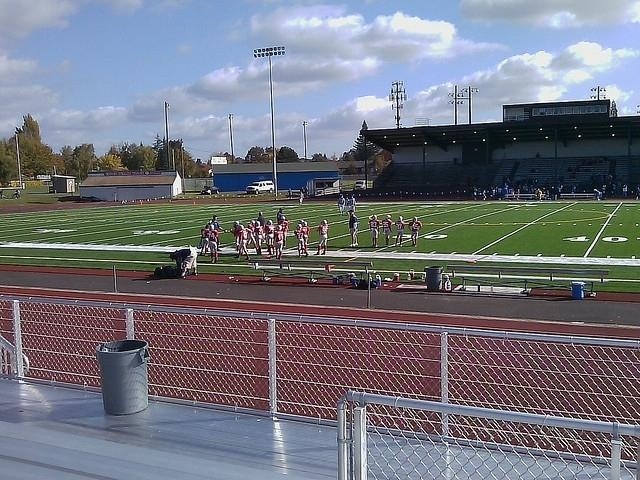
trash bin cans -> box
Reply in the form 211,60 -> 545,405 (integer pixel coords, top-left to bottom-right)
96,339 -> 152,415
424,265 -> 443,291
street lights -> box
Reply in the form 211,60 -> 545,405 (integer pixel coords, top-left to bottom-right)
228,113 -> 234,163
389,80 -> 407,128
253,45 -> 286,197
301,120 -> 308,159
165,101 -> 170,170
447,85 -> 480,125
590,85 -> 607,100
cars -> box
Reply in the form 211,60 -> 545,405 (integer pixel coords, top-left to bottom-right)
246,180 -> 274,195
201,186 -> 220,195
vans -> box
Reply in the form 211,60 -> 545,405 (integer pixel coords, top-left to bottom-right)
355,180 -> 367,190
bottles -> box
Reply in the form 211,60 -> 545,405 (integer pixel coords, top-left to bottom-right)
394,273 -> 399,281
409,269 -> 414,278
376,275 -> 382,287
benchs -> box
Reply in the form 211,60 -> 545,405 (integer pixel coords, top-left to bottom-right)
249,258 -> 373,284
444,264 -> 612,295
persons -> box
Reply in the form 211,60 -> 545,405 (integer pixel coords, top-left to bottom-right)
348,210 -> 422,247
338,194 -> 356,216
536,152 -> 540,158
582,156 -> 609,166
200,208 -> 328,264
0,189 -> 20,200
169,248 -> 198,279
298,191 -> 304,206
473,166 -> 640,202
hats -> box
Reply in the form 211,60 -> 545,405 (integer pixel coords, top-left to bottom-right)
170,250 -> 177,262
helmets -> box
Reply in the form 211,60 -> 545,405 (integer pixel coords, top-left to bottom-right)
232,219 -> 327,230
371,214 -> 418,221
